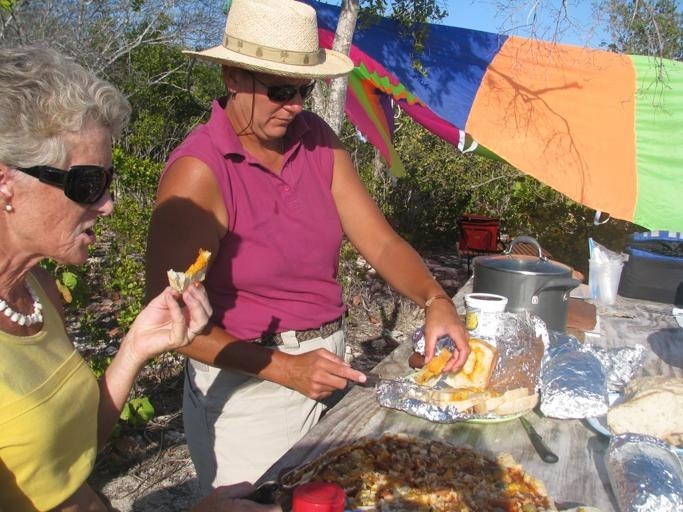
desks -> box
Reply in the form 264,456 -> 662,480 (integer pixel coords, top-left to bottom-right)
254,275 -> 683,512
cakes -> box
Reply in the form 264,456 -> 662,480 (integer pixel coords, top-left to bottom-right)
284,433 -> 559,511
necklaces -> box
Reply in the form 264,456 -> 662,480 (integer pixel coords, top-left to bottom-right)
1,278 -> 43,327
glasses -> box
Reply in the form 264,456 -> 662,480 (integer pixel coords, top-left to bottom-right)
245,69 -> 316,103
2,158 -> 114,205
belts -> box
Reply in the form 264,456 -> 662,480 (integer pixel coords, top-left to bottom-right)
240,310 -> 349,346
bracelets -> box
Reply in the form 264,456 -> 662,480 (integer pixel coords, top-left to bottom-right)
423,294 -> 455,315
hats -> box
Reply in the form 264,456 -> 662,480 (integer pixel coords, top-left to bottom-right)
181,0 -> 355,81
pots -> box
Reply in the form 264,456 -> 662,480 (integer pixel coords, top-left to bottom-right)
472,234 -> 581,333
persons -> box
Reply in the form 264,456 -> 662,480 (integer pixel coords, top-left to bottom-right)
143,0 -> 472,495
1,37 -> 286,512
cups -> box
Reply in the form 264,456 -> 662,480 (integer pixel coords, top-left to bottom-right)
463,293 -> 508,336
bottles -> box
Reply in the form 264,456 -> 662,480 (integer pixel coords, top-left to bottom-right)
291,486 -> 346,512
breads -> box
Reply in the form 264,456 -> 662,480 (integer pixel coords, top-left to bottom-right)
605,373 -> 683,443
164,248 -> 211,293
409,336 -> 538,419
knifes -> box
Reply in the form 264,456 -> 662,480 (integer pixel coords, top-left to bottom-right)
520,415 -> 559,463
